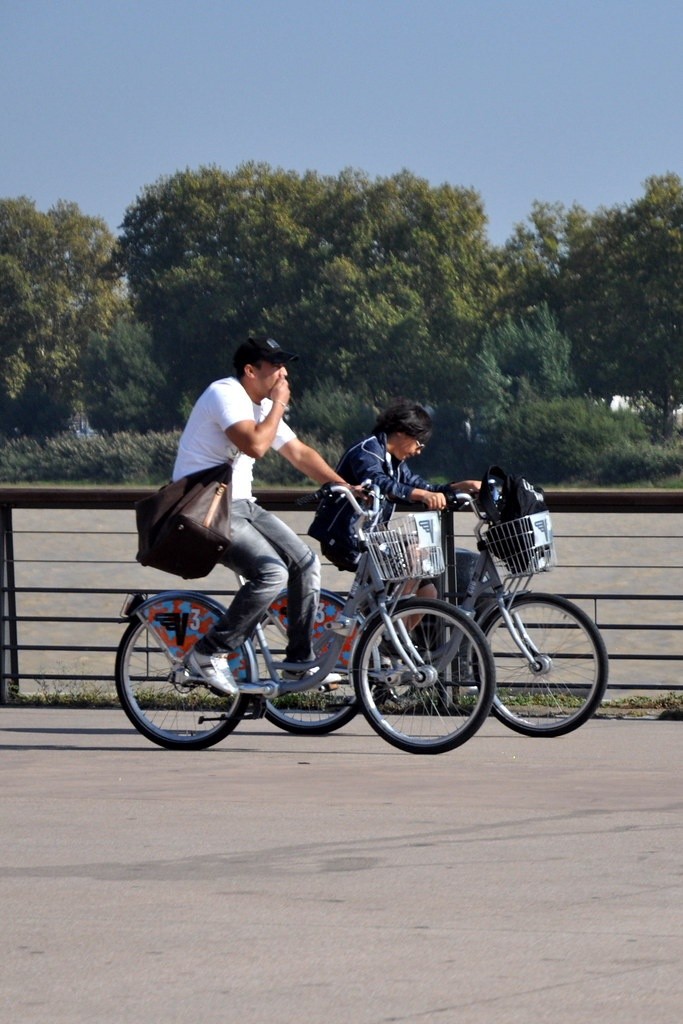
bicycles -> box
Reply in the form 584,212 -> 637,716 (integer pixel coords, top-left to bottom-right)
251,479 -> 611,736
112,479 -> 496,754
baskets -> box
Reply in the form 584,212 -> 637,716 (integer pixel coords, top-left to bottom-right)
360,512 -> 445,581
482,510 -> 557,576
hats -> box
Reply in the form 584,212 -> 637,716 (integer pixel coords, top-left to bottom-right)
234,339 -> 300,364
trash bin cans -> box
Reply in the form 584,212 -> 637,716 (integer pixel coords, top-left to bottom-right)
379,547 -> 479,686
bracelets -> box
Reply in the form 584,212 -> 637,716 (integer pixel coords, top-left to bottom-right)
274,400 -> 286,408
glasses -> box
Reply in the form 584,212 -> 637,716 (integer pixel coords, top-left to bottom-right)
412,438 -> 426,452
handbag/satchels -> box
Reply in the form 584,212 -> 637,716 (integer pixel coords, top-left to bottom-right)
135,462 -> 232,578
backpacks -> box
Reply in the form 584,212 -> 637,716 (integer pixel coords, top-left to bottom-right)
482,467 -> 544,571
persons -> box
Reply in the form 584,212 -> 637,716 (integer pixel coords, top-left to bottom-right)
307,404 -> 485,682
172,333 -> 369,696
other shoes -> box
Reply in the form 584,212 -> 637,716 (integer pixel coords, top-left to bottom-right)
379,635 -> 428,661
373,685 -> 405,705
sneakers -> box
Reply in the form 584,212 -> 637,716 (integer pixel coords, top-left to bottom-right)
280,662 -> 341,687
185,647 -> 238,697
